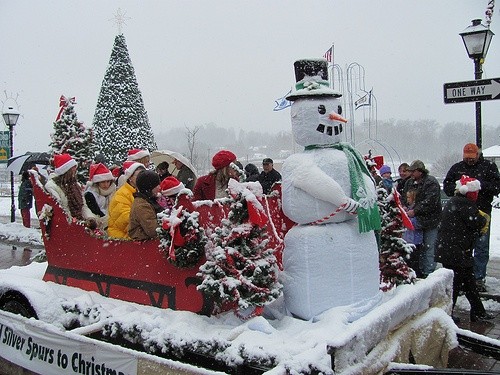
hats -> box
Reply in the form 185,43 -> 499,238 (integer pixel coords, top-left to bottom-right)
462,143 -> 477,159
380,165 -> 391,176
112,168 -> 120,181
284,58 -> 343,101
406,159 -> 425,171
89,163 -> 114,183
157,161 -> 169,170
161,176 -> 185,197
212,150 -> 236,170
123,161 -> 146,179
263,158 -> 273,166
455,175 -> 481,195
128,149 -> 150,160
136,170 -> 163,194
54,154 -> 78,176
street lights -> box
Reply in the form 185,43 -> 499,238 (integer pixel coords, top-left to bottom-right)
1,106 -> 21,223
458,19 -> 495,154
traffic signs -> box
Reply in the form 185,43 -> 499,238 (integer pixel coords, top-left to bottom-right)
443,77 -> 500,104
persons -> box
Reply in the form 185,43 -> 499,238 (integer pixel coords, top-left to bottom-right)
281,59 -> 379,322
366,144 -> 500,322
19,149 -> 282,242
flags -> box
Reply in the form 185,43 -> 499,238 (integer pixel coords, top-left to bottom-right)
273,91 -> 291,111
322,47 -> 333,62
354,91 -> 372,110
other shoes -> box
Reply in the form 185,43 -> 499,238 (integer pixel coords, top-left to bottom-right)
475,280 -> 487,292
416,272 -> 428,279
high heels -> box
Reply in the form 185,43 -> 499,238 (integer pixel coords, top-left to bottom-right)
451,316 -> 460,323
470,308 -> 495,322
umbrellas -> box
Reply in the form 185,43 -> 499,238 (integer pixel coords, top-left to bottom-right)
149,150 -> 198,178
6,153 -> 53,174
